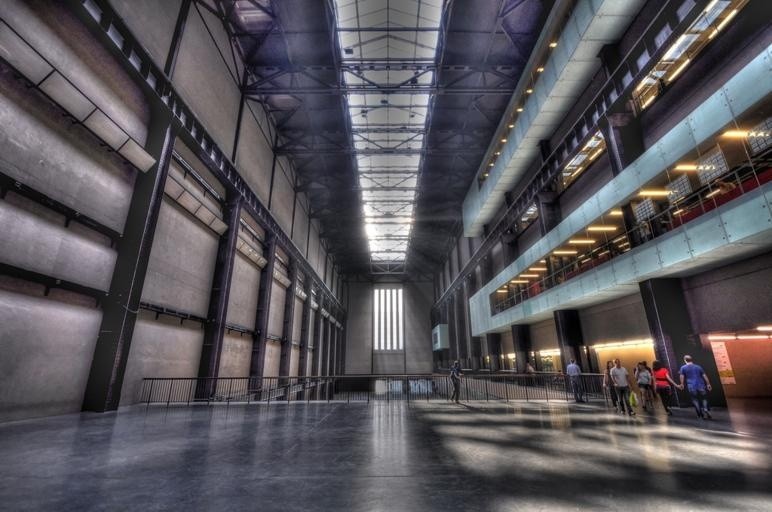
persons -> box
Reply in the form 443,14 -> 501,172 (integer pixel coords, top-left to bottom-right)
657,77 -> 670,95
679,355 -> 712,420
633,220 -> 651,243
652,360 -> 682,416
567,359 -> 586,403
449,361 -> 464,404
715,178 -> 736,195
525,363 -> 534,374
602,358 -> 656,416
530,280 -> 540,296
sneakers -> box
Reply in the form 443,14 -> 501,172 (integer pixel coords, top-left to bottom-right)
576,398 -> 584,403
697,408 -> 712,420
613,404 -> 673,416
